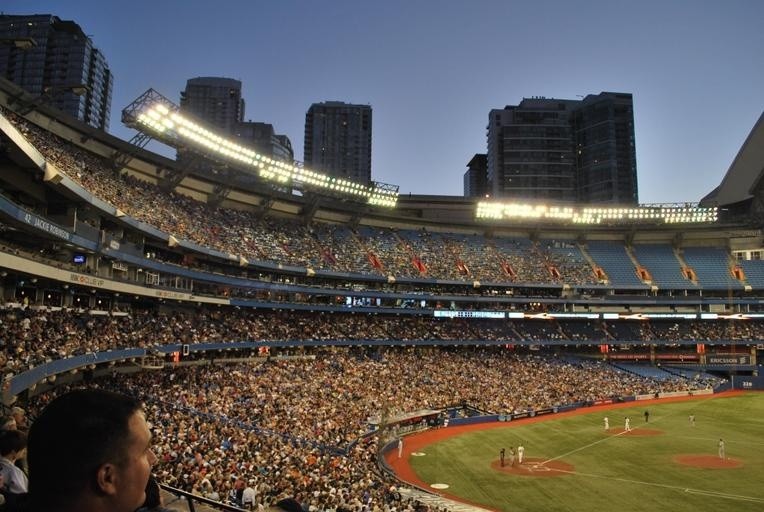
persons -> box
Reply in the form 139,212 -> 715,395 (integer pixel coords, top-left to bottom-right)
2,102 -> 764,511
